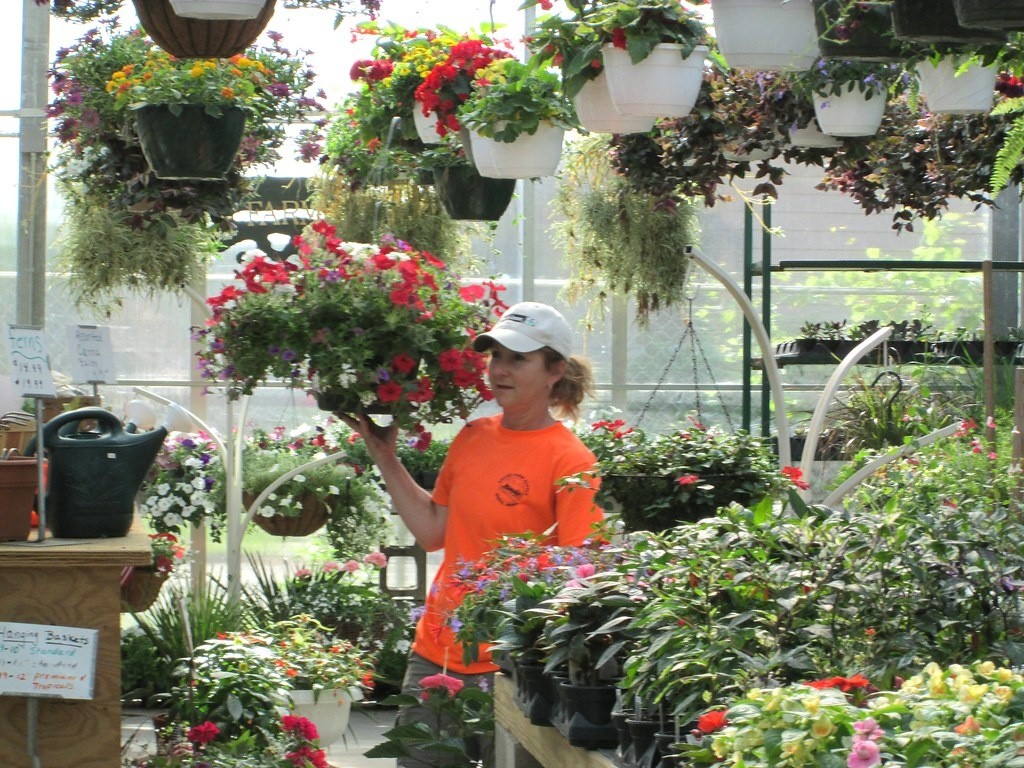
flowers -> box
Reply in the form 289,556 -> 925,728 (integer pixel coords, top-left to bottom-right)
348,18 -> 506,140
573,69 -> 657,134
362,673 -> 497,768
561,0 -> 731,76
786,56 -> 912,110
753,70 -> 822,134
146,531 -> 200,581
414,39 -> 521,138
189,219 -> 510,452
285,552 -> 387,638
105,49 -> 275,119
415,132 -> 468,172
209,446 -> 400,561
41,21 -> 331,175
554,405 -> 811,519
223,414 -> 454,475
389,520 -> 659,684
455,57 -> 589,143
519,14 -> 603,101
137,632 -> 336,768
660,417 -> 1024,768
136,433 -> 215,536
214,613 -> 387,707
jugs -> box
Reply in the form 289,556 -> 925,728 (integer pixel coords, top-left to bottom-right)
22,406 -> 167,539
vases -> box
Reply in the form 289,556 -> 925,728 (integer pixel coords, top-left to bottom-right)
509,647 -> 709,768
309,332 -> 422,414
126,175 -> 176,212
411,99 -> 441,143
464,119 -> 566,178
788,110 -> 844,148
609,473 -> 753,530
712,0 -> 819,72
917,52 -> 997,114
600,42 -> 709,119
953,0 -> 1024,30
288,688 -> 351,748
0,395 -> 102,543
721,136 -> 774,161
813,0 -> 916,63
131,0 -> 277,58
169,0 -> 266,20
811,79 -> 888,137
136,103 -> 246,180
431,164 -> 515,221
891,0 -> 1008,45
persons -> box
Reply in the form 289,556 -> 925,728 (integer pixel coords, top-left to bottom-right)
331,301 -> 612,768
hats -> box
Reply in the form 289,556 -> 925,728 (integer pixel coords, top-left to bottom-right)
475,302 -> 573,362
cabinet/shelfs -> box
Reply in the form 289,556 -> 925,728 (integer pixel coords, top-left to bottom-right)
741,195 -> 1024,463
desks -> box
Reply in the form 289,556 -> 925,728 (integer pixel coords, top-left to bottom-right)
0,500 -> 153,768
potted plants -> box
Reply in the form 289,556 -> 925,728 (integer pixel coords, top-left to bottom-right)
794,321 -> 821,352
909,320 -> 933,355
819,318 -> 847,355
933,324 -> 1024,366
881,320 -> 914,356
846,320 -> 881,358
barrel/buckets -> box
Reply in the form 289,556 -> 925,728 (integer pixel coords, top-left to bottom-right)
0,456 -> 42,543
